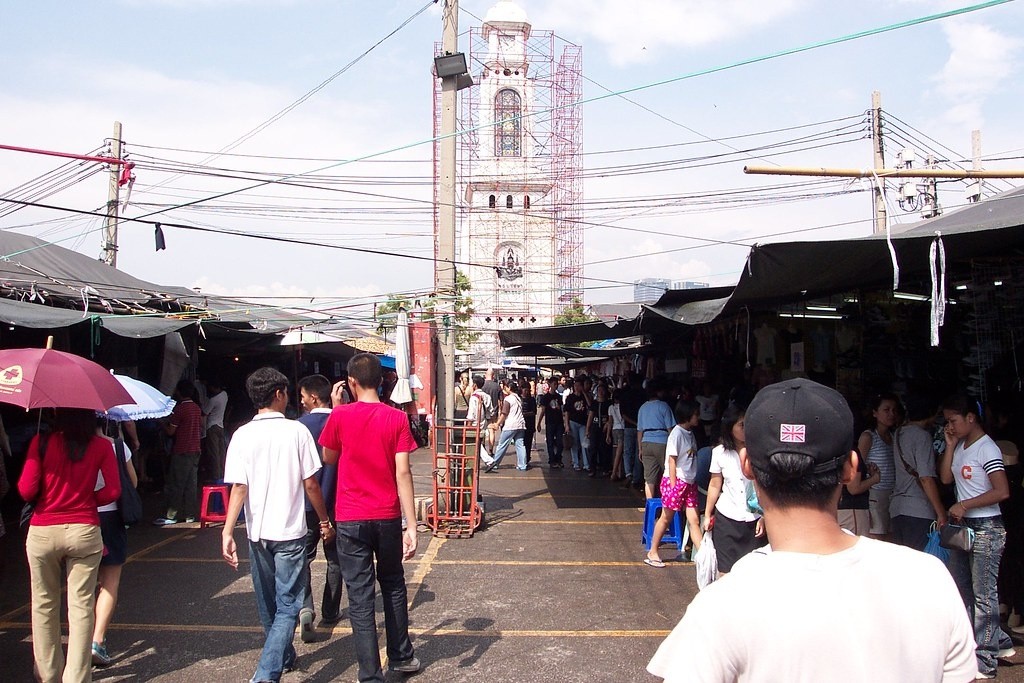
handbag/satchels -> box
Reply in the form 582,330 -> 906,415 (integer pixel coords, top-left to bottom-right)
938,516 -> 976,552
117,437 -> 144,524
924,520 -> 969,584
563,431 -> 573,449
694,531 -> 719,592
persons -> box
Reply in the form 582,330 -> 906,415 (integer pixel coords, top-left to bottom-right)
91,409 -> 142,666
151,379 -> 203,527
297,374 -> 342,643
318,351 -> 427,683
754,322 -> 858,371
201,377 -> 229,485
16,405 -> 122,683
646,376 -> 981,683
836,392 -> 1018,682
221,367 -> 337,683
448,373 -> 769,579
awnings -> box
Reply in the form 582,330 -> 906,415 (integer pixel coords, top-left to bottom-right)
491,186 -> 1024,417
0,226 -> 366,364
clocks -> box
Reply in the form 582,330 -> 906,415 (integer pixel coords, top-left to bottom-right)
500,37 -> 515,51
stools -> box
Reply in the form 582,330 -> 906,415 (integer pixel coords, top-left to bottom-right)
200,486 -> 230,529
641,499 -> 682,553
214,479 -> 246,524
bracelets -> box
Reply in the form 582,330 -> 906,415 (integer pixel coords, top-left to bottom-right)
318,517 -> 331,528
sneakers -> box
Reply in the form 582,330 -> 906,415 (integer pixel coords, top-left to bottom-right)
92,636 -> 110,664
389,658 -> 420,670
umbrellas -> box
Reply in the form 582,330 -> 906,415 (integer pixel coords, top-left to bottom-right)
390,302 -> 415,413
0,336 -> 180,437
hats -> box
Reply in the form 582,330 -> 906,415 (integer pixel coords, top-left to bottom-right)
743,378 -> 853,475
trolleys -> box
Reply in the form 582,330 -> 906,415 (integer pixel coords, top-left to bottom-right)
426,395 -> 482,536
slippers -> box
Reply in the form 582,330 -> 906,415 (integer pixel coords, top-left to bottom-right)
644,559 -> 666,568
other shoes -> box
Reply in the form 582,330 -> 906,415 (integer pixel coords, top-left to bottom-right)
998,647 -> 1016,658
323,611 -> 344,624
299,612 -> 316,642
975,672 -> 995,679
552,462 -> 633,489
484,462 -> 496,473
153,518 -> 176,525
186,517 -> 194,523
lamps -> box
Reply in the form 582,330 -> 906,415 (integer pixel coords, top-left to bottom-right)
954,278 -> 1003,290
806,302 -> 839,312
843,295 -> 924,306
893,292 -> 957,305
776,309 -> 842,320
